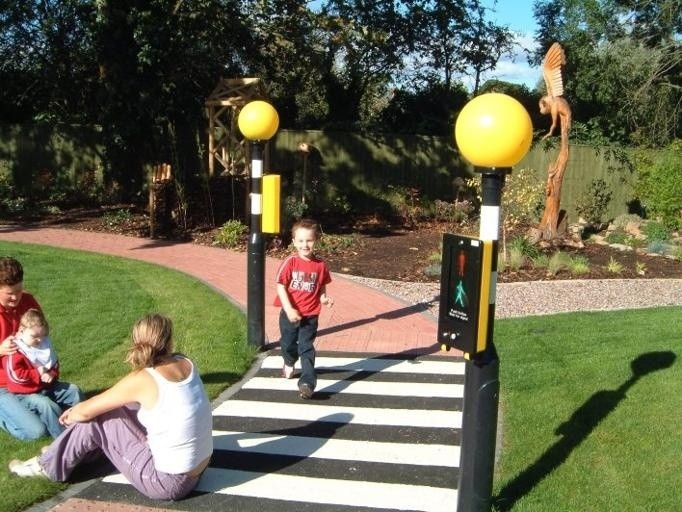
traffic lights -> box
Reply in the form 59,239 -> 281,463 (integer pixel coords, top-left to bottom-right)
436,232 -> 487,359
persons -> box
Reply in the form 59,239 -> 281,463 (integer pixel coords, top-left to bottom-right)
272,218 -> 334,400
0,256 -> 82,443
9,313 -> 214,502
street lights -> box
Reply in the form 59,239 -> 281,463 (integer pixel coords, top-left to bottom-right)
234,98 -> 280,350
451,88 -> 538,504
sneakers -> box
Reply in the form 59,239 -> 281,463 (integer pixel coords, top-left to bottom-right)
283,364 -> 295,379
297,377 -> 315,400
8,444 -> 52,479
